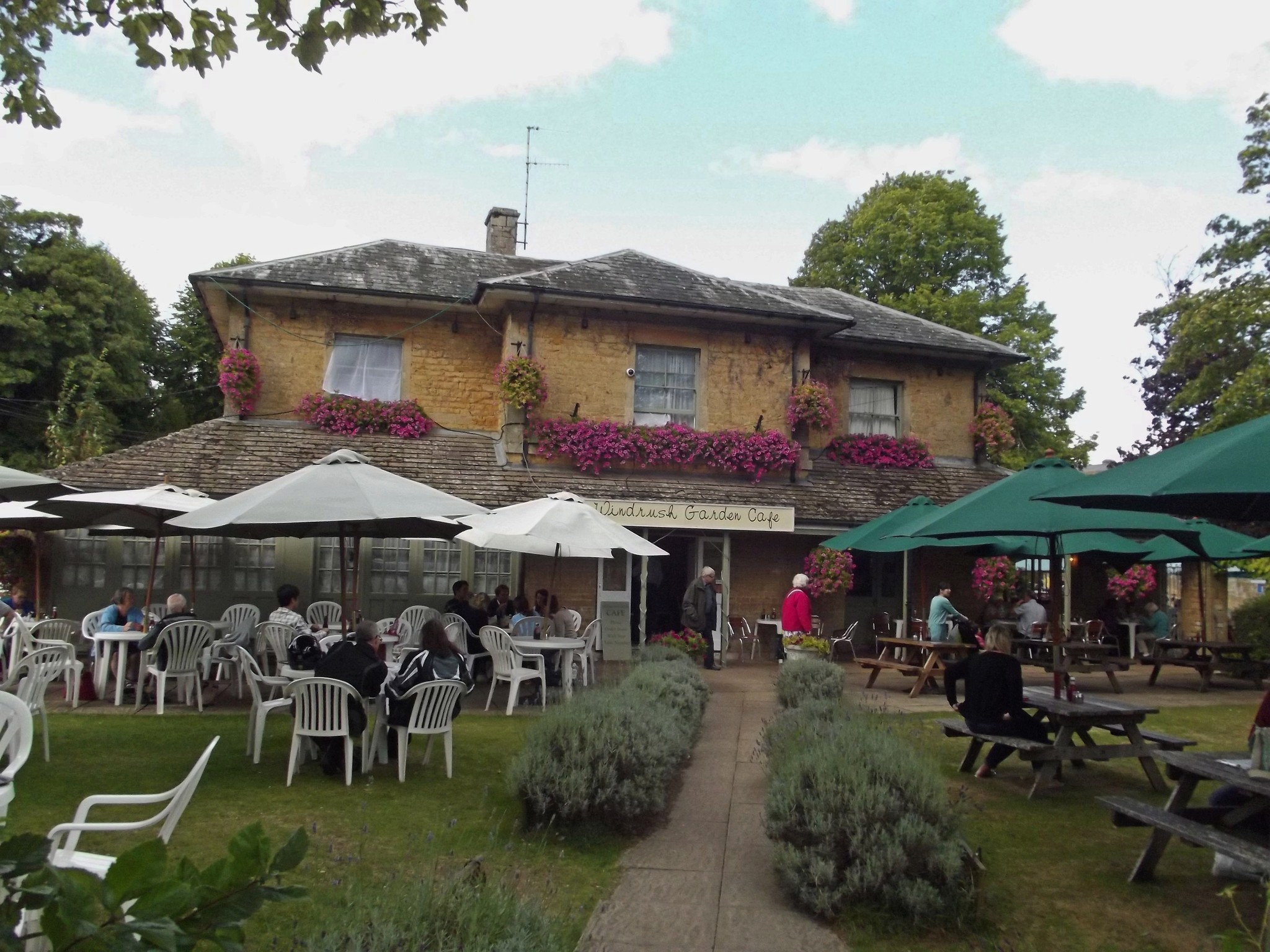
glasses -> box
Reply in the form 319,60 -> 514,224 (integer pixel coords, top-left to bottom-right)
376,635 -> 383,641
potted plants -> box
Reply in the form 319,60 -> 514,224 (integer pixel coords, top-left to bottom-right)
782,634 -> 829,662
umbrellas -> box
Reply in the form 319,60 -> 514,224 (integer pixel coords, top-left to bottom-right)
269,519 -> 472,609
1229,535 -> 1270,554
455,491 -> 670,641
818,496 -> 1029,660
162,449 -> 491,642
0,466 -> 219,633
878,410 -> 1270,772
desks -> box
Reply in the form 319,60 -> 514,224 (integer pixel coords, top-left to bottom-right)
0,619 -> 81,677
861,638 -> 976,698
893,619 -> 955,659
1129,748 -> 1270,883
1119,621 -> 1137,658
511,636 -> 585,701
93,631 -> 146,705
279,658 -> 401,765
749,619 -> 782,661
991,619 -> 1021,656
1150,638 -> 1264,693
204,619 -> 232,680
1009,639 -> 1123,695
811,615 -> 1121,663
379,635 -> 399,661
960,686 -> 1167,797
307,621 -> 348,630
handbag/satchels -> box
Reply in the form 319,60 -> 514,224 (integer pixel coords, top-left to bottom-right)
64,671 -> 96,700
287,633 -> 321,669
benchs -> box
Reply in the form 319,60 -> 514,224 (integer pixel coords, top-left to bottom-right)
859,637 -> 1270,883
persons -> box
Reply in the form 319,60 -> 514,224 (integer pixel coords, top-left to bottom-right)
681,566 -> 722,670
1135,600 -> 1170,657
929,582 -> 980,670
0,584 -> 36,676
943,624 -> 1040,777
781,574 -> 812,653
1208,689 -> 1270,796
1004,576 -> 1047,657
100,588 -> 209,703
445,581 -> 578,685
269,585 -> 329,641
1098,599 -> 1129,656
290,619 -> 474,772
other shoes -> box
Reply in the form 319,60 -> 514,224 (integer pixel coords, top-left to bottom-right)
704,663 -> 721,670
317,751 -> 337,773
125,682 -> 134,690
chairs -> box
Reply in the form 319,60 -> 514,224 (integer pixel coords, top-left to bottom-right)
0,690 -> 219,952
725,615 -> 761,662
1,601 -> 601,787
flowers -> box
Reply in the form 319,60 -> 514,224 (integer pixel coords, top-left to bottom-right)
652,629 -> 706,661
218,346 -> 1016,482
803,547 -> 1157,595
